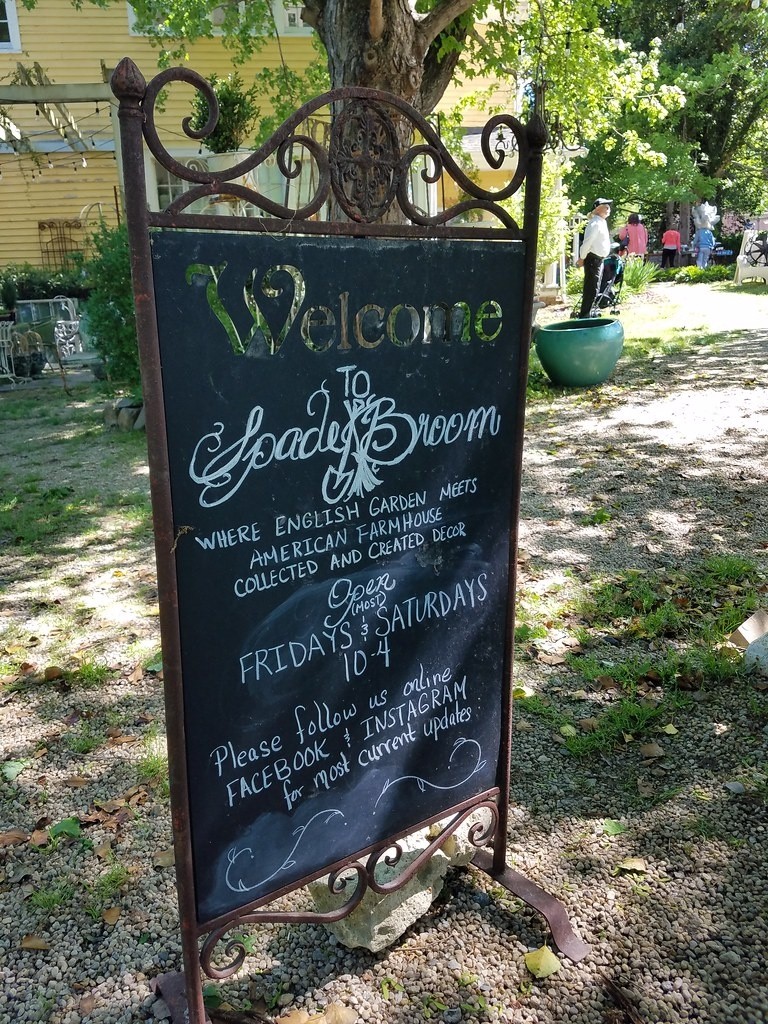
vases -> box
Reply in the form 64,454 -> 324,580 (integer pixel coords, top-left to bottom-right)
534,318 -> 624,388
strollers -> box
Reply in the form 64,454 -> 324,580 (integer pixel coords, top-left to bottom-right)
570,232 -> 629,318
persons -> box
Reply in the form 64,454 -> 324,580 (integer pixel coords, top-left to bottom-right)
693,228 -> 720,271
619,214 -> 648,261
660,224 -> 680,268
577,198 -> 613,319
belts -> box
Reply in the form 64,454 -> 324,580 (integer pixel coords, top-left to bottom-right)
589,253 -> 602,260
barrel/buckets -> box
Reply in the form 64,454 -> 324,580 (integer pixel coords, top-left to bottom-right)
534,316 -> 625,386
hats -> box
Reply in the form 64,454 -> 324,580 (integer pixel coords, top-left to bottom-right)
593,198 -> 613,209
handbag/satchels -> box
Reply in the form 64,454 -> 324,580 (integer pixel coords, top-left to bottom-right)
620,237 -> 629,250
694,247 -> 699,254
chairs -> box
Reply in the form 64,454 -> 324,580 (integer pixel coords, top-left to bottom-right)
734,229 -> 768,286
